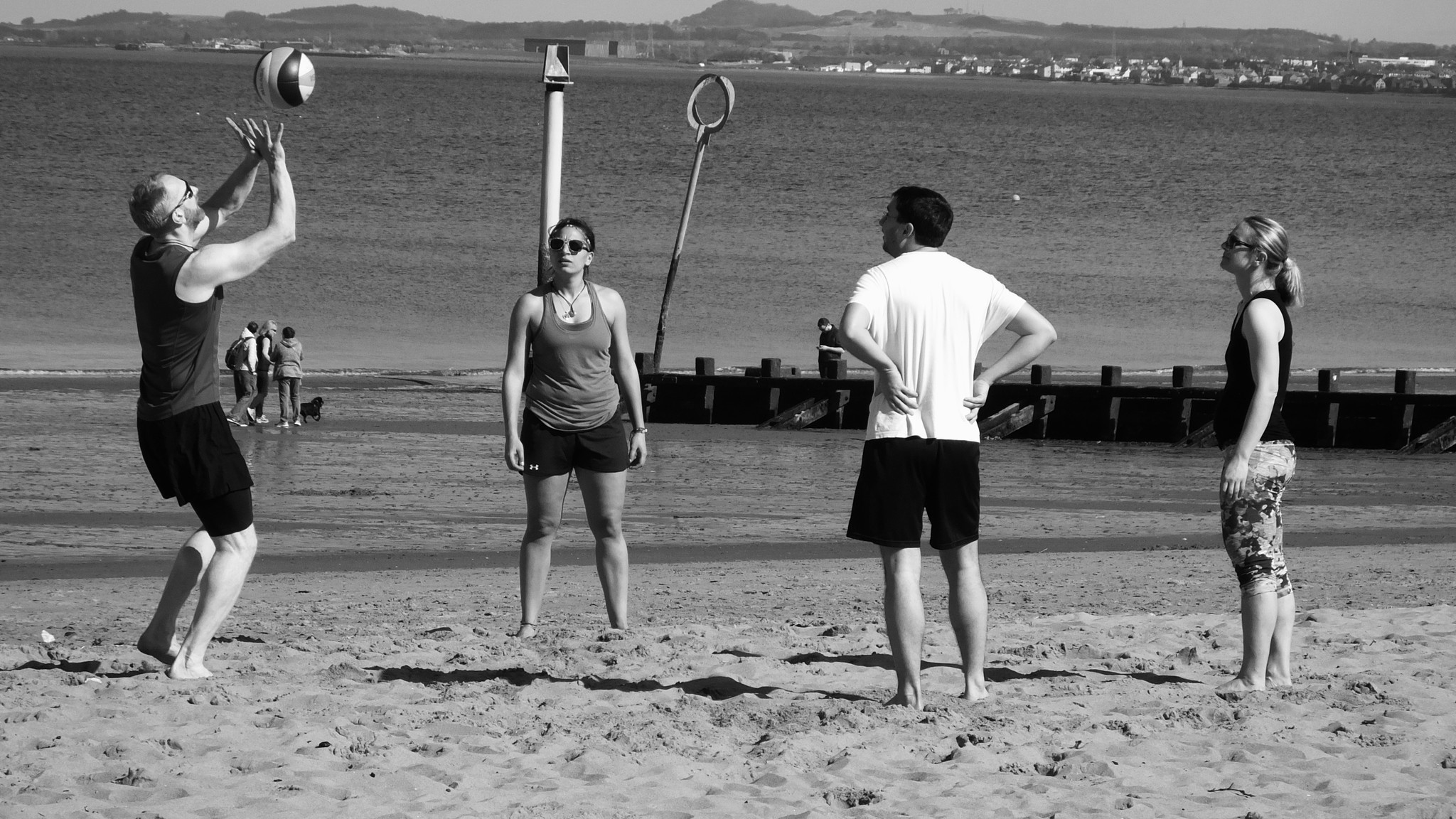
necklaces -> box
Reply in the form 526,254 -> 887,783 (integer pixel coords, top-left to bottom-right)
550,284 -> 586,319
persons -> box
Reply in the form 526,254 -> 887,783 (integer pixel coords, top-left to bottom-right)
815,319 -> 846,379
226,320 -> 304,428
502,218 -> 648,638
836,184 -> 1060,710
129,116 -> 297,683
1219,214 -> 1306,694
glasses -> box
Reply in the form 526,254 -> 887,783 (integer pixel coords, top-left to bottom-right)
273,329 -> 276,332
162,178 -> 193,222
548,237 -> 591,252
819,323 -> 828,331
1226,233 -> 1262,254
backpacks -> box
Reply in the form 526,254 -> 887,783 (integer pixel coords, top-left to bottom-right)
225,337 -> 256,371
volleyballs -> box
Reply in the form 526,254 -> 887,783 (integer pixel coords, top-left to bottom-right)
252,45 -> 316,111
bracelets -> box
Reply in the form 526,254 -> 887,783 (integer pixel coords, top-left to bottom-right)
629,427 -> 648,441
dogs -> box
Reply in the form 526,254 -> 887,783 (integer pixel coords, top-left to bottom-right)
300,396 -> 324,423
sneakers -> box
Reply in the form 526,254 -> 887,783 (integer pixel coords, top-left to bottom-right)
247,407 -> 255,421
255,414 -> 269,423
226,415 -> 249,426
274,422 -> 289,428
294,421 -> 301,426
